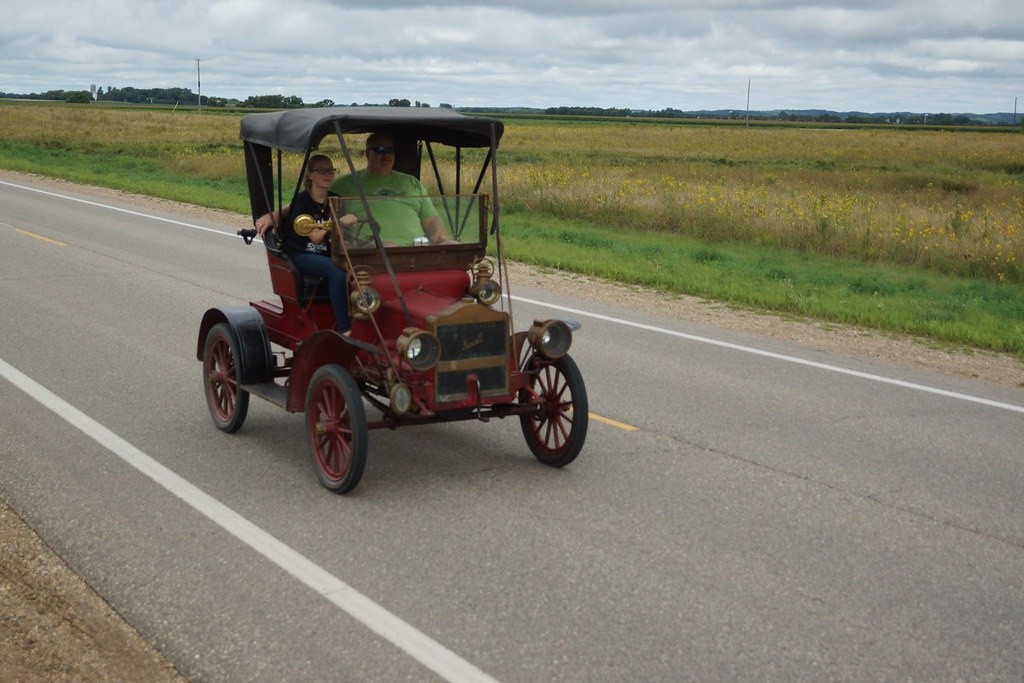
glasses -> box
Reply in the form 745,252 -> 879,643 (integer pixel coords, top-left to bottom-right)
369,145 -> 396,154
312,167 -> 336,176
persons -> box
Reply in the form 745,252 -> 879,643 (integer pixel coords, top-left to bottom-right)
255,134 -> 460,248
280,155 -> 357,337
